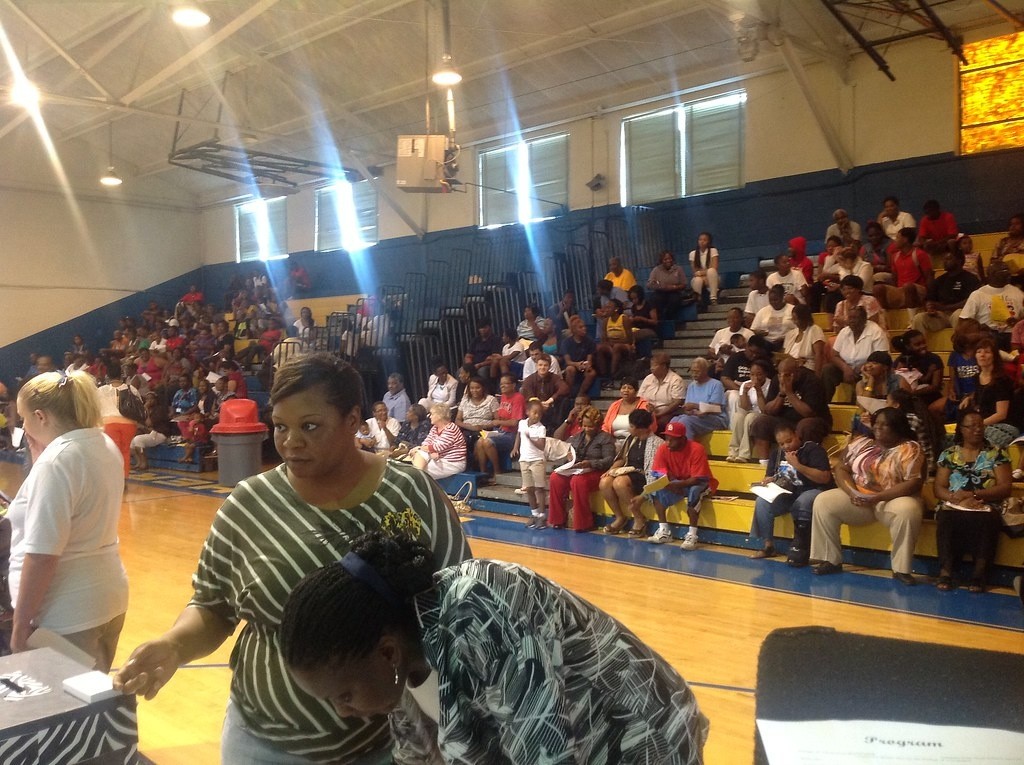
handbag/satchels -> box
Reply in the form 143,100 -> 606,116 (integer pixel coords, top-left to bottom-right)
446,480 -> 473,516
119,384 -> 148,424
608,465 -> 636,476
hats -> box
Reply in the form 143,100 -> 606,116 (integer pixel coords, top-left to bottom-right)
169,318 -> 179,326
660,422 -> 687,437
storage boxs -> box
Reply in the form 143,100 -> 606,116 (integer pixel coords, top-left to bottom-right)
0,647 -> 139,765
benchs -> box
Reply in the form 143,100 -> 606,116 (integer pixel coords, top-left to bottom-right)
130,227 -> 1024,568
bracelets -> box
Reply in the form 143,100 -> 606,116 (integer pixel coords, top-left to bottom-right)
863,386 -> 873,391
778,392 -> 786,398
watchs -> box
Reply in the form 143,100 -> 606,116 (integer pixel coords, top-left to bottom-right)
973,490 -> 977,499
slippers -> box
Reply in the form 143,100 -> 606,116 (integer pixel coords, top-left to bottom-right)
937,576 -> 953,592
967,580 -> 986,593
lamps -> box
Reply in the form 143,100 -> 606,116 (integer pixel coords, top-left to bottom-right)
431,0 -> 464,87
100,109 -> 122,185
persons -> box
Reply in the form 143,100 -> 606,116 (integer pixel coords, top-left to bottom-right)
357,192 -> 1024,592
279,506 -> 708,765
112,350 -> 471,765
4,370 -> 128,674
0,268 -> 313,472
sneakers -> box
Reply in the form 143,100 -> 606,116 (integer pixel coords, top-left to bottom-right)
680,532 -> 698,549
647,527 -> 675,544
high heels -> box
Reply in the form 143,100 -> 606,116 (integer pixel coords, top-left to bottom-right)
543,522 -> 567,530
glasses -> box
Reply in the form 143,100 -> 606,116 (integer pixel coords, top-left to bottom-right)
961,423 -> 987,433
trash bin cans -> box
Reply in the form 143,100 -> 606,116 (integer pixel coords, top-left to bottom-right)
208,399 -> 269,487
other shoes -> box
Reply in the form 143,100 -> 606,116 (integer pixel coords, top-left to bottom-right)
786,555 -> 809,567
526,516 -> 547,529
748,547 -> 777,558
627,522 -> 647,539
812,560 -> 843,574
605,519 -> 628,534
515,487 -> 527,494
726,456 -> 747,464
131,464 -> 149,472
893,570 -> 916,586
